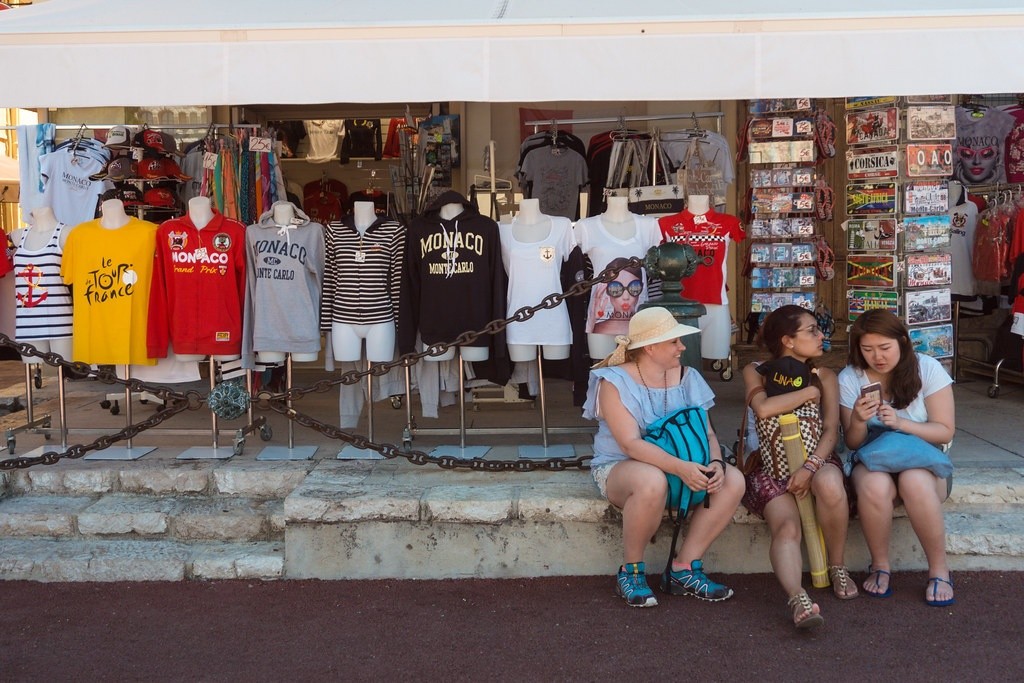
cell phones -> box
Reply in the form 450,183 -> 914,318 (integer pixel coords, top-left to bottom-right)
860,382 -> 883,409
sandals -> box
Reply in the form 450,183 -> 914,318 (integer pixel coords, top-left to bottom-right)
829,564 -> 859,600
925,574 -> 953,606
863,562 -> 892,598
787,586 -> 825,628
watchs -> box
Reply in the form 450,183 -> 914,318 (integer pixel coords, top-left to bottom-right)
711,459 -> 726,475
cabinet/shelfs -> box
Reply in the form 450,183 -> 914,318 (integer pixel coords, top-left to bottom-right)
735,98 -> 954,377
101,123 -> 182,221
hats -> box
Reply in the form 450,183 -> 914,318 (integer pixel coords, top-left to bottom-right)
589,307 -> 701,369
89,124 -> 194,212
767,356 -> 810,410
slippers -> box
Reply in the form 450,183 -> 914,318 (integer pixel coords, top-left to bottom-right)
734,110 -> 840,353
741,240 -> 768,279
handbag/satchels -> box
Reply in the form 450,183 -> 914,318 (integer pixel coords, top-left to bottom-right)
847,423 -> 952,500
603,134 -> 726,216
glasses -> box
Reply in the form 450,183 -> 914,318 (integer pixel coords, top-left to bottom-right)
787,324 -> 820,333
606,279 -> 644,297
957,146 -> 997,161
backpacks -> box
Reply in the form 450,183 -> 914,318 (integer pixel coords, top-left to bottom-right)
734,385 -> 823,485
643,404 -> 711,511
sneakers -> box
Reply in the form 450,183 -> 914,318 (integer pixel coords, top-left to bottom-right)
660,551 -> 733,601
615,562 -> 658,608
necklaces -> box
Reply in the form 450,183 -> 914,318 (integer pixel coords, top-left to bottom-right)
636,361 -> 667,420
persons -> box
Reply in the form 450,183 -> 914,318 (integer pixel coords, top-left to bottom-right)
498,199 -> 577,361
62,198 -> 159,367
656,194 -> 747,359
241,200 -> 334,370
575,197 -> 664,360
400,189 -> 507,361
146,196 -> 248,362
582,306 -> 745,606
321,201 -> 406,362
836,309 -> 955,605
11,207 -> 73,363
735,305 -> 859,630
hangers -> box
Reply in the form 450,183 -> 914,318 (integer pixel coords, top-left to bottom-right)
546,113 -> 710,154
67,122 -> 92,160
195,121 -> 239,155
283,167 -> 381,197
989,184 -> 1024,217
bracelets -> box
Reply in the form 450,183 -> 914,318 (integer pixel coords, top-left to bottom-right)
801,455 -> 826,472
